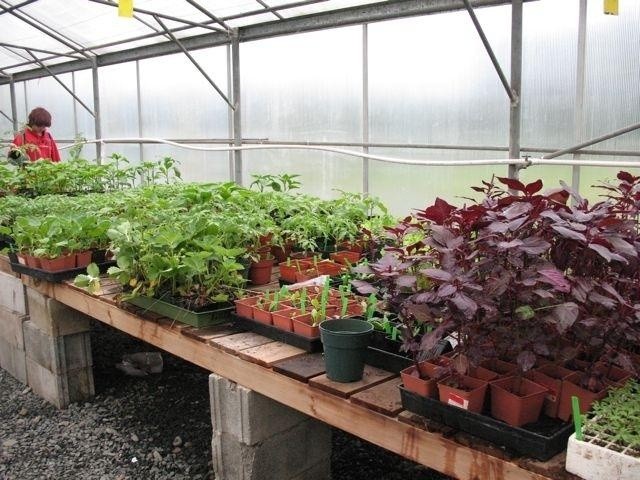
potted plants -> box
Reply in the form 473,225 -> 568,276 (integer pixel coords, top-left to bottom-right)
122,185 -> 244,326
282,299 -> 310,308
288,288 -> 306,297
318,213 -> 348,260
306,285 -> 325,293
289,217 -> 321,263
270,225 -> 292,263
351,173 -> 640,480
0,194 -> 121,271
245,216 -> 271,260
236,296 -> 270,320
304,294 -> 335,305
323,289 -> 352,298
293,310 -> 327,339
0,160 -> 170,198
248,240 -> 275,287
258,292 -> 286,302
322,299 -> 352,319
281,261 -> 313,284
253,302 -> 289,326
314,261 -> 344,272
298,267 -> 326,283
332,250 -> 359,263
273,308 -> 309,333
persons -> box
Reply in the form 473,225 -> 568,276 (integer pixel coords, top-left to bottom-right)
8,108 -> 61,162
